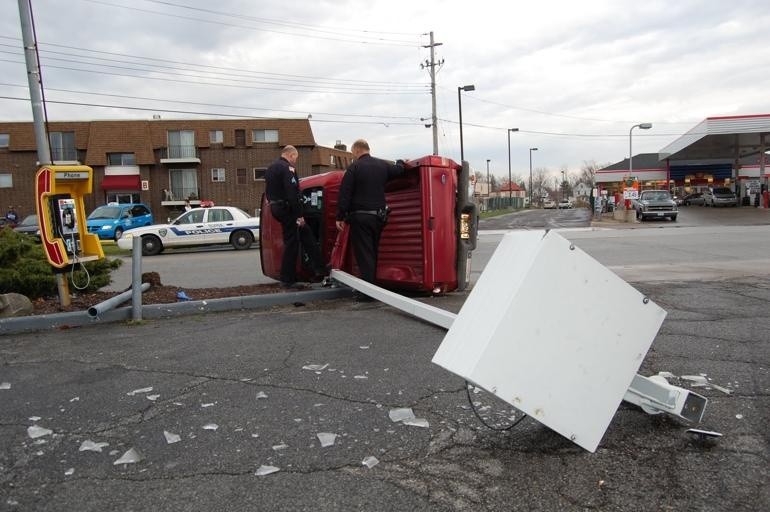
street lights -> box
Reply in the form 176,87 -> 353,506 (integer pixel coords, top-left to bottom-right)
487,158 -> 492,198
561,170 -> 564,200
456,83 -> 475,162
530,147 -> 538,204
508,127 -> 520,206
629,122 -> 653,179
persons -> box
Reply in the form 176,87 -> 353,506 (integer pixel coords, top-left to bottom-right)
263,144 -> 335,293
334,138 -> 406,302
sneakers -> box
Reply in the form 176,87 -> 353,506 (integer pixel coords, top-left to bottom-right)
316,263 -> 332,276
352,289 -> 372,301
280,281 -> 304,289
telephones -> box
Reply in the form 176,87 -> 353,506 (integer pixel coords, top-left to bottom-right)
54,199 -> 79,237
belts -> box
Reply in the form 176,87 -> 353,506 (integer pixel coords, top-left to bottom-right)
269,199 -> 286,203
351,210 -> 378,215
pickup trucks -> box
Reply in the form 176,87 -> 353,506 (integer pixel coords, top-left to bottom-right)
631,187 -> 679,221
259,151 -> 479,295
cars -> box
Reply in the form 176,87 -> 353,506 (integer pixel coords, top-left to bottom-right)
544,200 -> 557,209
119,200 -> 261,255
14,212 -> 43,243
559,199 -> 573,209
0,216 -> 17,231
85,199 -> 152,241
673,185 -> 738,208
